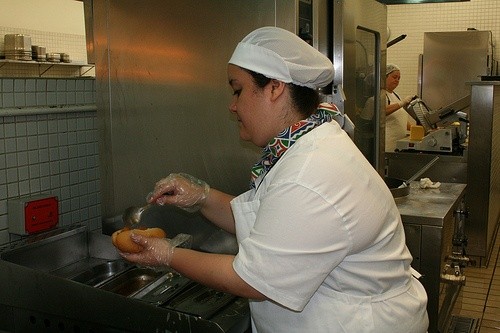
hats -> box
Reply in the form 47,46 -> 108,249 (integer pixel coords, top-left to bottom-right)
385,63 -> 400,76
228,26 -> 335,91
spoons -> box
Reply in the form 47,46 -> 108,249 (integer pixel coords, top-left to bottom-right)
121,202 -> 155,227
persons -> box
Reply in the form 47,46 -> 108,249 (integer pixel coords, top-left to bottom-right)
384,64 -> 414,153
120,26 -> 429,333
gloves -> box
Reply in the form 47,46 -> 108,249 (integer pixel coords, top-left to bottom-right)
398,96 -> 412,108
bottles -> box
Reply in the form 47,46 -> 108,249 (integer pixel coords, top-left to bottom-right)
32,45 -> 46,62
4,34 -> 33,61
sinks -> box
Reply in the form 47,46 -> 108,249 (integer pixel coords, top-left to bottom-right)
1,227 -> 193,297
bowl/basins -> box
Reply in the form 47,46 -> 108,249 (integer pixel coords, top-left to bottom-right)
47,53 -> 61,62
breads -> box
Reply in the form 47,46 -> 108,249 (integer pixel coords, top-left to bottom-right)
110,226 -> 167,253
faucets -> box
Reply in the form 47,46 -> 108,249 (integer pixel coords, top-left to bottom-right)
442,260 -> 466,286
406,98 -> 431,114
447,238 -> 471,263
